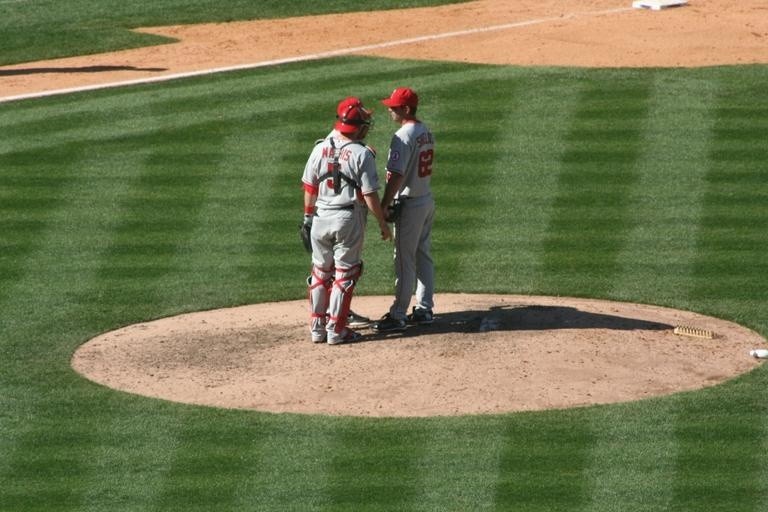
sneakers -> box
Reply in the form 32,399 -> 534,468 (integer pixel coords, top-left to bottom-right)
370,312 -> 433,332
310,306 -> 370,345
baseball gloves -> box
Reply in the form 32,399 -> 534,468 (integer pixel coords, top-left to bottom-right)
300,222 -> 313,254
381,197 -> 403,223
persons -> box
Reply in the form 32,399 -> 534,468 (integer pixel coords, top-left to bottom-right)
301,96 -> 392,345
370,87 -> 435,331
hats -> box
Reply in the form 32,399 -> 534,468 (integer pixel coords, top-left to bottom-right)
381,87 -> 418,108
335,97 -> 374,133
